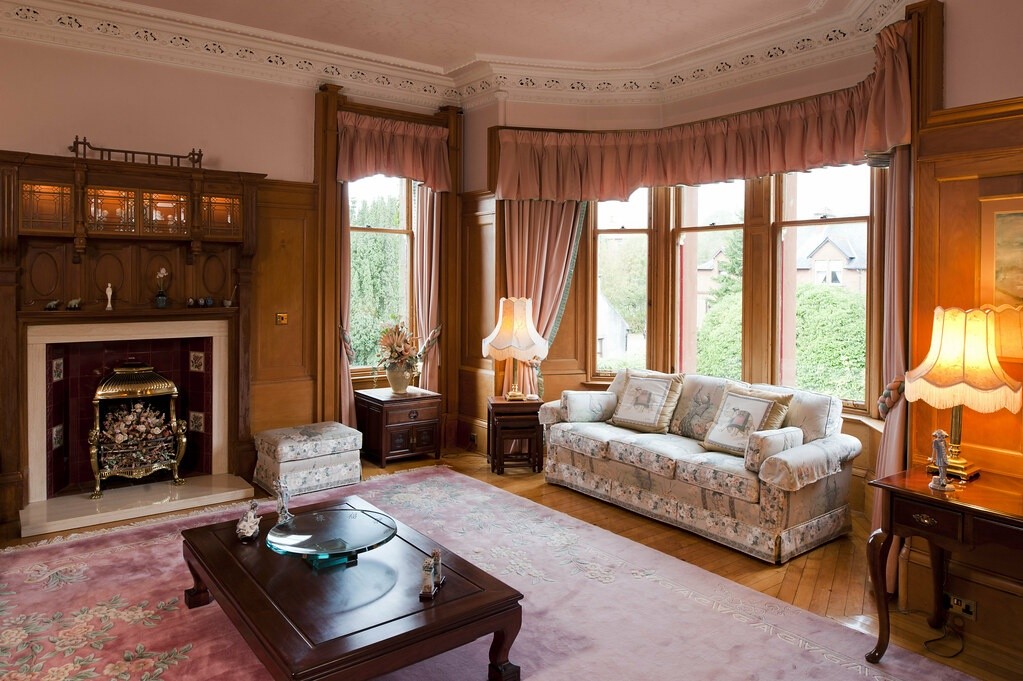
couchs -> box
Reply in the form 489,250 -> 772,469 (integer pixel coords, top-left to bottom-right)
538,367 -> 862,563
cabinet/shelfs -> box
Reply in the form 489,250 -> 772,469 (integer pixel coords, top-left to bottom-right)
0,149 -> 267,255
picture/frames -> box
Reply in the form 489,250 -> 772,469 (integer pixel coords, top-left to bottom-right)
978,195 -> 1023,308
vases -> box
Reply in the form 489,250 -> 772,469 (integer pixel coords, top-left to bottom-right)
386,362 -> 414,393
154,290 -> 168,308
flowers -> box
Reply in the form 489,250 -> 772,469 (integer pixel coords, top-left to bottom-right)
378,323 -> 421,378
155,267 -> 168,289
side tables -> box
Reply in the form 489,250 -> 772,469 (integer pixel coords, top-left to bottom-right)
487,394 -> 546,475
354,385 -> 443,468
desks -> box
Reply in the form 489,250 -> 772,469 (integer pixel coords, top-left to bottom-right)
865,461 -> 1023,663
181,494 -> 524,681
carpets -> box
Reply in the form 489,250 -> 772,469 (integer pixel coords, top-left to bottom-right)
0,462 -> 979,681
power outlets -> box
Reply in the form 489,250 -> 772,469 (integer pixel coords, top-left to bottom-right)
950,596 -> 977,619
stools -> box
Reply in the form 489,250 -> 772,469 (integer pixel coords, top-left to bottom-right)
251,420 -> 363,497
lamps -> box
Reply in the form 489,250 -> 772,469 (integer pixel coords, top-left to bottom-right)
906,306 -> 1023,483
482,294 -> 549,401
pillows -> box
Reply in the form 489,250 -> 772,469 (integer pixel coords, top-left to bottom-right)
606,365 -> 685,435
560,389 -> 617,420
697,377 -> 794,457
744,425 -> 804,472
